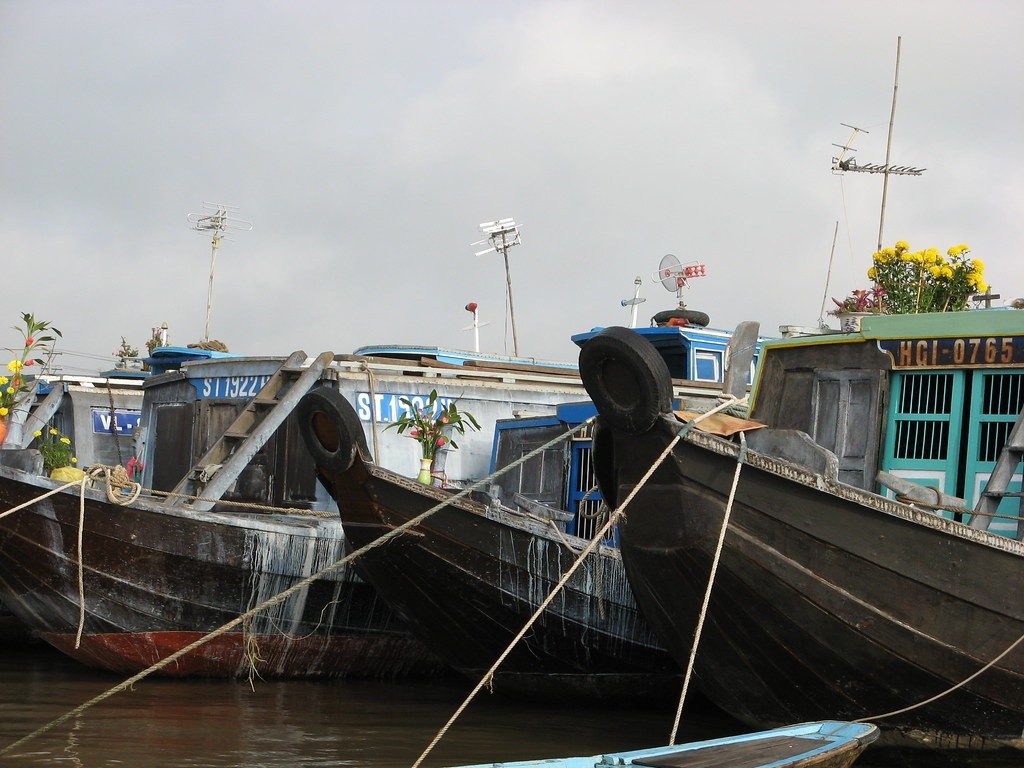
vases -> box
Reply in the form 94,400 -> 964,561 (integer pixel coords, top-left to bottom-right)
114,362 -> 141,371
839,311 -> 874,334
416,458 -> 433,485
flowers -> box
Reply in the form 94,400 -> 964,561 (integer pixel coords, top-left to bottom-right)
33,429 -> 78,477
826,286 -> 880,316
868,239 -> 987,314
379,388 -> 482,462
0,311 -> 63,427
110,336 -> 139,362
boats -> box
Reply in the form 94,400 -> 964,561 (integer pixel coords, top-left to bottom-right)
0,30 -> 1024,768
